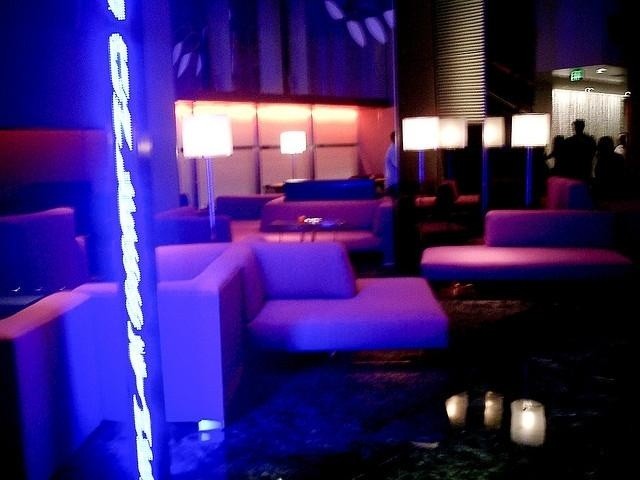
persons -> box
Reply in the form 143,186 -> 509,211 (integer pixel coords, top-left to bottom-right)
384,129 -> 397,197
546,117 -> 628,196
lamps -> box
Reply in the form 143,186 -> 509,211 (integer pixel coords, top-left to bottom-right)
183,109 -> 234,243
401,113 -> 552,210
280,130 -> 306,179
